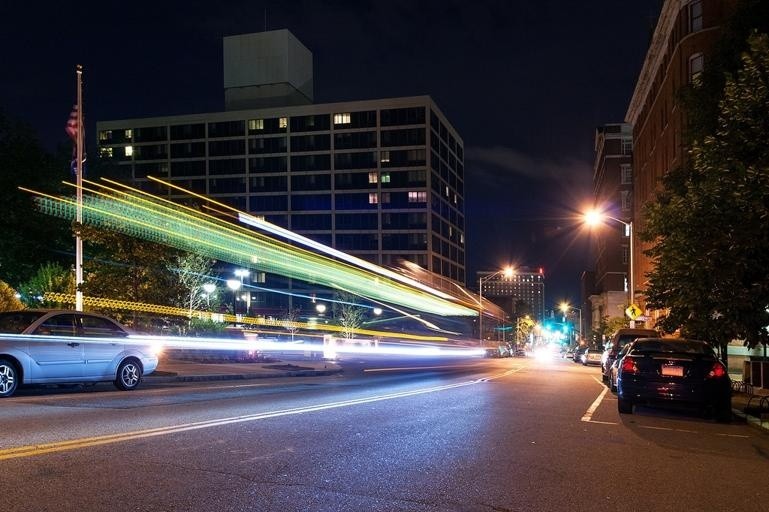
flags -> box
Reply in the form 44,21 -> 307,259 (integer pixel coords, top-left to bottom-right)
64,92 -> 87,178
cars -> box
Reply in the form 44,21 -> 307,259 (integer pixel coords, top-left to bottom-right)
486,348 -> 529,359
559,328 -> 733,420
1,309 -> 161,397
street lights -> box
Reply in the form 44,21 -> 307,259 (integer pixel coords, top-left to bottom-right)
204,283 -> 216,309
227,280 -> 241,326
586,211 -> 636,329
235,269 -> 250,292
561,304 -> 582,346
479,268 -> 513,345
